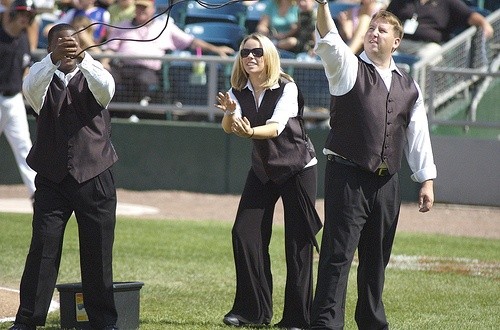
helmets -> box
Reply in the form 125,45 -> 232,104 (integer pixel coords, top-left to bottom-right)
10,0 -> 36,15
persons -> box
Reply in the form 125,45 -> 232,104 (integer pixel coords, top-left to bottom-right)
312,0 -> 437,330
0,0 -> 37,205
213,32 -> 323,330
102,0 -> 235,123
7,23 -> 118,330
243,0 -> 495,65
27,0 -> 136,61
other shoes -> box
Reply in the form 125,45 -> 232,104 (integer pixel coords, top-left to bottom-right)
290,327 -> 304,330
223,315 -> 241,326
10,325 -> 36,330
104,325 -> 118,330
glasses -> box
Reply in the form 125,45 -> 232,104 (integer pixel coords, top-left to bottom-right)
240,48 -> 263,58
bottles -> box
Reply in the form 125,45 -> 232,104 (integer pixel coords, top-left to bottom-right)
189,48 -> 207,85
402,12 -> 419,35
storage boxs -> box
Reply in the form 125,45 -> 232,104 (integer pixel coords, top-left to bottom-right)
55,281 -> 144,330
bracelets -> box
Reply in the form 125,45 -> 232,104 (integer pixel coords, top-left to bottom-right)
248,128 -> 255,138
224,110 -> 235,115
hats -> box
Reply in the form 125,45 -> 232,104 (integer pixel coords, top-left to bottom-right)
135,0 -> 153,8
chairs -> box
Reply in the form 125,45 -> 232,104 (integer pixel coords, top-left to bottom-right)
37,0 -> 490,68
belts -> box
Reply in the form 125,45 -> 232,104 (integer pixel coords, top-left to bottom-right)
328,154 -> 389,176
